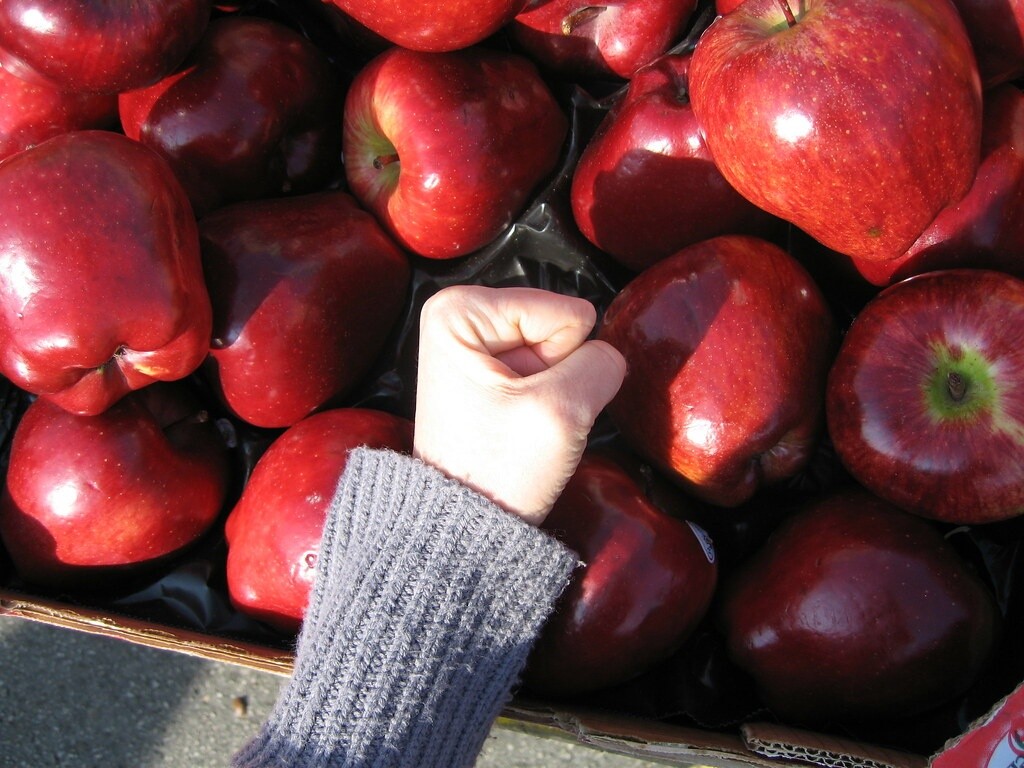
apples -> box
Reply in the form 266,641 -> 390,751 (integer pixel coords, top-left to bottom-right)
0,0 -> 1024,745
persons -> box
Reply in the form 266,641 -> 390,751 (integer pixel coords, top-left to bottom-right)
229,286 -> 628,768
